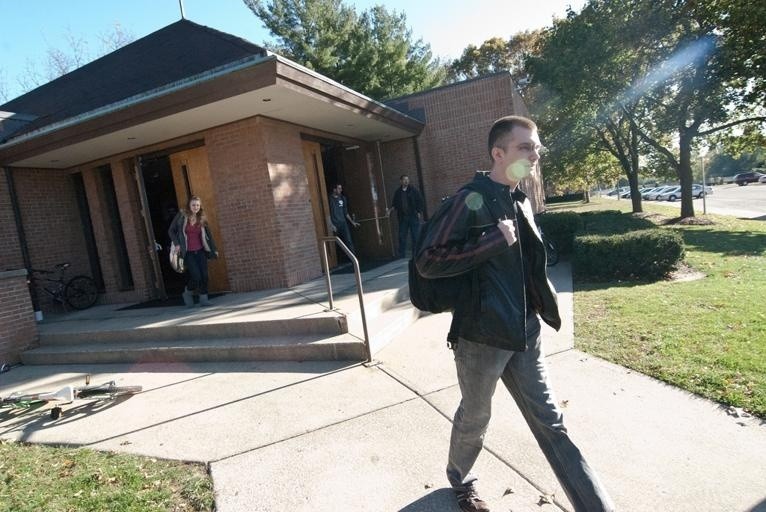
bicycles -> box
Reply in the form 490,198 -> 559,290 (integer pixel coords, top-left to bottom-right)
536,215 -> 560,268
6,261 -> 99,313
0,363 -> 142,420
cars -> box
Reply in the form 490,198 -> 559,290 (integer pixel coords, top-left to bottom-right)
602,170 -> 715,203
699,171 -> 766,187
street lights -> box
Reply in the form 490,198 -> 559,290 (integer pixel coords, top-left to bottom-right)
697,139 -> 709,215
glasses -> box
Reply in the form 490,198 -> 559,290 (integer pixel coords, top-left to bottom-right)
497,144 -> 542,154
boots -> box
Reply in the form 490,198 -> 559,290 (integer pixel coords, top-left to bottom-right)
182,286 -> 195,308
198,294 -> 214,306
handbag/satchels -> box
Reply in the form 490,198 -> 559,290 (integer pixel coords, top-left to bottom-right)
169,240 -> 186,274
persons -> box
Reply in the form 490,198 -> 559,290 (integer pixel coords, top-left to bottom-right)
385,175 -> 424,258
415,116 -> 613,512
168,196 -> 218,308
328,183 -> 361,257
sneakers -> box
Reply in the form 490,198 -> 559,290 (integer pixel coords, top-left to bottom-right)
454,488 -> 489,510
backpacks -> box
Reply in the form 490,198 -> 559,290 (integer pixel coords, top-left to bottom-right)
408,186 -> 508,351
